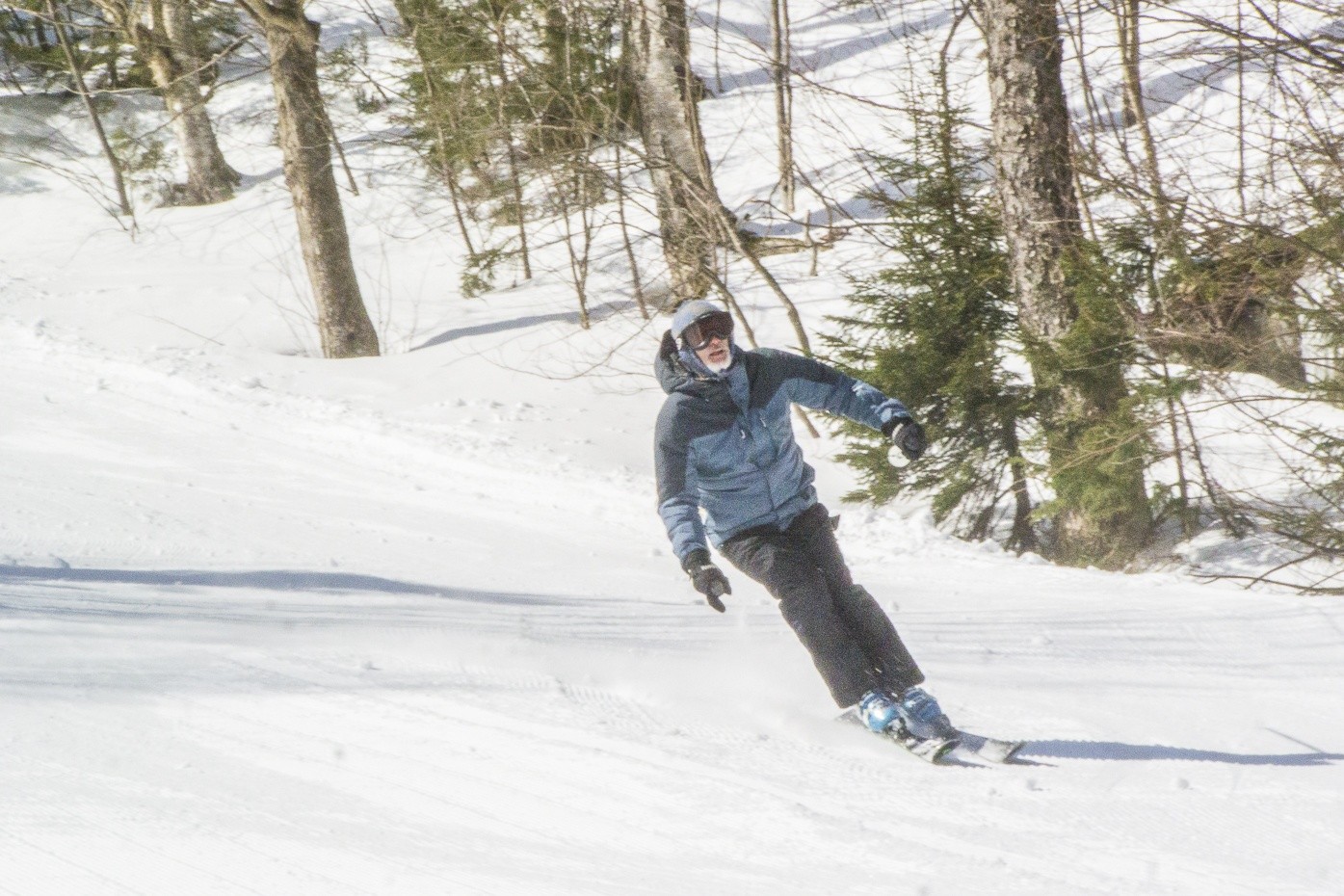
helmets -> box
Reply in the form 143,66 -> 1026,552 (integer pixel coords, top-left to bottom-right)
670,299 -> 722,340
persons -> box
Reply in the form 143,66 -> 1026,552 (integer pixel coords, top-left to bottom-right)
653,299 -> 954,739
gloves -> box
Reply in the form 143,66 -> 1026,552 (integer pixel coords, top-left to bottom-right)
881,415 -> 925,461
683,547 -> 732,612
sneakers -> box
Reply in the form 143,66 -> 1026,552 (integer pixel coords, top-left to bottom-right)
854,688 -> 900,732
892,685 -> 941,722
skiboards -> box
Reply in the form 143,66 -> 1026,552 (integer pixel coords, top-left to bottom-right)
835,707 -> 1027,764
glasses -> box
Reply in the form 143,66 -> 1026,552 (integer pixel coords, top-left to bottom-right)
678,310 -> 735,350
